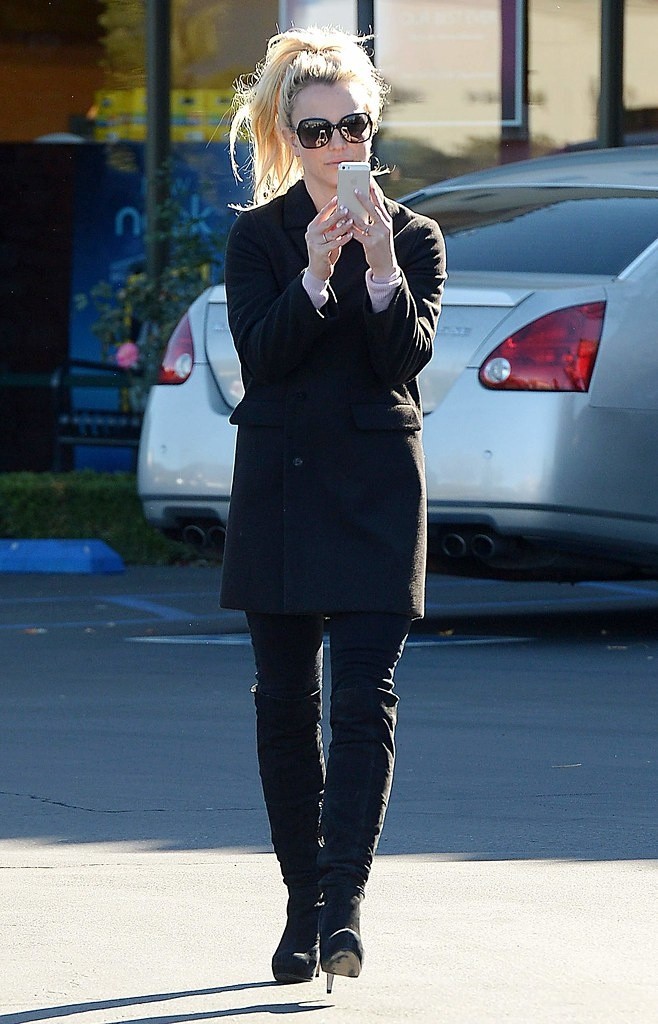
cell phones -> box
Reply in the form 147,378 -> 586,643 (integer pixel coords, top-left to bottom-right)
336,162 -> 370,231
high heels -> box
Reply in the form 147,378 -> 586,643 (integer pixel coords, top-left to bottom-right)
271,916 -> 319,981
317,903 -> 364,994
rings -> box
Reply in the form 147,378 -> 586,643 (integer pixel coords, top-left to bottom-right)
322,233 -> 328,243
365,226 -> 371,235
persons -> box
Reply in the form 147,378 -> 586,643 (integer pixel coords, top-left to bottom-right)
224,24 -> 445,999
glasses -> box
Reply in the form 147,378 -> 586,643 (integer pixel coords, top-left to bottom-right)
293,112 -> 373,149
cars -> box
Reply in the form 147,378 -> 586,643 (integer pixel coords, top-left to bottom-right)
133,142 -> 658,615
